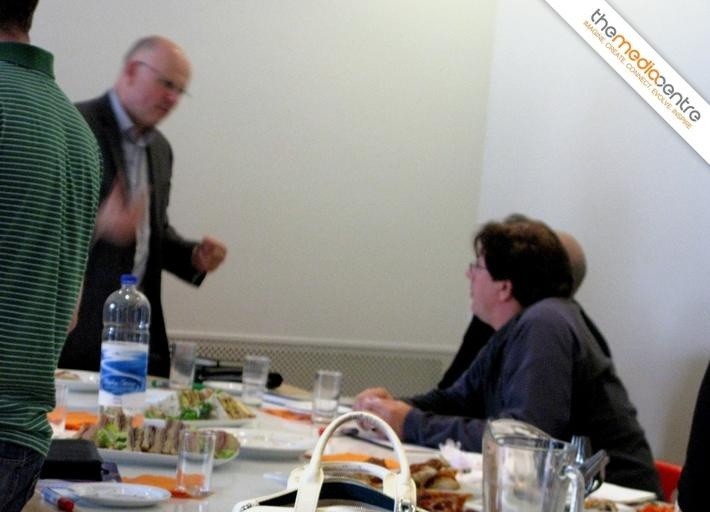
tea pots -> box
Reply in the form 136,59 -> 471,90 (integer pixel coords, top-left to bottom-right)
481,417 -> 587,512
537,437 -> 610,512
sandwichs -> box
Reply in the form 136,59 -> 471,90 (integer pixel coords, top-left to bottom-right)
72,387 -> 258,461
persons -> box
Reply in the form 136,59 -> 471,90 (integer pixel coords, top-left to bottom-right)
357,214 -> 670,506
55,36 -> 228,382
1,1 -> 107,512
434,230 -> 614,391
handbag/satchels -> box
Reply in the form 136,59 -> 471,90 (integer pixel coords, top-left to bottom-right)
233,411 -> 427,511
40,438 -> 109,482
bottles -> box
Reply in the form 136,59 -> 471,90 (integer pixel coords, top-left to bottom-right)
42,487 -> 74,512
100,274 -> 151,408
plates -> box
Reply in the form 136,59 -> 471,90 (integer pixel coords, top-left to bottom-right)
585,481 -> 656,505
288,397 -> 355,419
127,401 -> 255,429
74,481 -> 171,506
226,430 -> 315,459
53,369 -> 101,393
97,436 -> 240,467
204,377 -> 246,397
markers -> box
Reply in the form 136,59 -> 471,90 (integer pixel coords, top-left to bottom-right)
39,487 -> 76,510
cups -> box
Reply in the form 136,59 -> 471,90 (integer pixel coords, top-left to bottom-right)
311,369 -> 345,422
174,430 -> 216,495
240,356 -> 270,405
169,340 -> 196,390
47,381 -> 69,436
